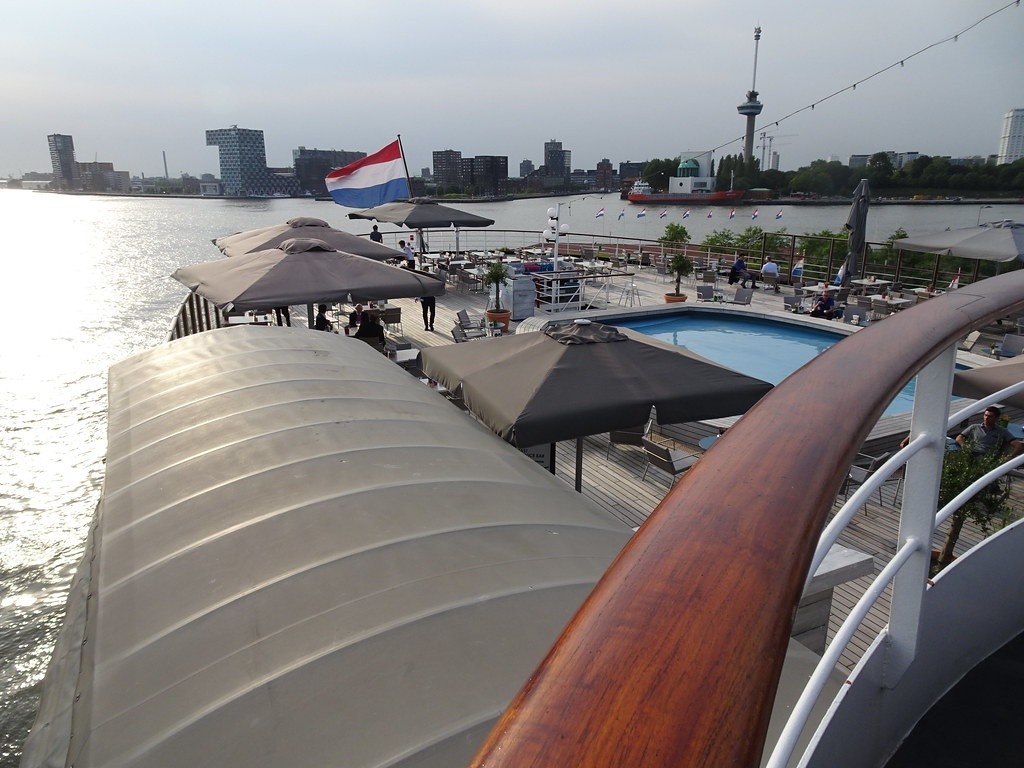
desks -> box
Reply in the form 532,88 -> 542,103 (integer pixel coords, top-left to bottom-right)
522,249 -> 550,254
698,435 -> 718,450
352,304 -> 397,330
229,315 -> 272,326
484,322 -> 505,337
866,294 -> 912,313
420,379 -> 447,392
851,279 -> 892,296
548,257 -> 579,260
388,348 -> 420,364
472,252 -> 492,267
464,269 -> 490,293
945,437 -> 960,450
424,254 -> 448,271
909,288 -> 946,296
324,327 -> 359,336
576,262 -> 613,275
439,260 -> 472,265
488,259 -> 520,264
801,285 -> 844,309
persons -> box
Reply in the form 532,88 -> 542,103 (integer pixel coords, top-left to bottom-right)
814,291 -> 834,312
761,256 -> 781,293
956,406 -> 1021,495
735,253 -> 760,289
350,302 -> 386,350
399,240 -> 416,270
273,305 -> 292,328
370,225 -> 383,244
315,303 -> 339,335
792,252 -> 803,276
414,266 -> 436,332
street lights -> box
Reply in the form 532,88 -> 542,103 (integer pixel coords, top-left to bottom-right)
542,202 -> 569,307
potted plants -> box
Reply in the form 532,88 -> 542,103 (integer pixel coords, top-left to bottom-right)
485,261 -> 512,331
664,254 -> 693,303
926,435 -> 1017,583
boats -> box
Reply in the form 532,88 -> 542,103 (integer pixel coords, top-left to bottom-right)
630,193 -> 746,205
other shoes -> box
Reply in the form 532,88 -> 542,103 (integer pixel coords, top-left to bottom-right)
751,285 -> 759,289
741,282 -> 746,288
424,325 -> 429,331
430,324 -> 434,331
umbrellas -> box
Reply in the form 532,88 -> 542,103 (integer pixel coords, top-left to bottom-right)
414,317 -> 777,492
348,196 -> 495,271
171,238 -> 436,328
844,178 -> 870,275
212,217 -> 413,261
893,220 -> 1024,275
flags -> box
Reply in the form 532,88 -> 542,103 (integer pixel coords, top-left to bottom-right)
595,207 -> 605,218
660,209 -> 667,219
728,207 -> 735,219
751,208 -> 758,220
707,210 -> 713,218
325,138 -> 410,208
637,208 -> 646,218
618,207 -> 625,221
775,208 -> 783,220
682,209 -> 690,219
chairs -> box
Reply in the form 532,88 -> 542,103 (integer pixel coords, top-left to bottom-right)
312,247 -> 1024,517
